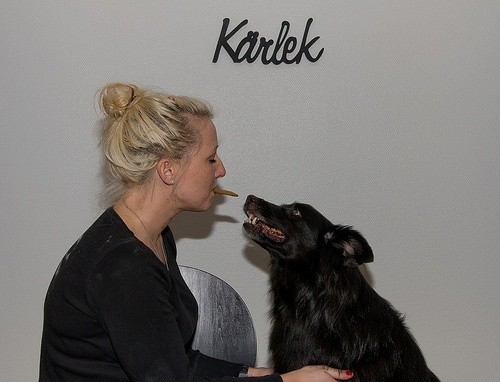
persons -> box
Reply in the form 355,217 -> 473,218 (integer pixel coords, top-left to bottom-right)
37,84 -> 354,382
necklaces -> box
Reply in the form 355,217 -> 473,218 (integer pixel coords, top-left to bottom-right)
120,199 -> 171,271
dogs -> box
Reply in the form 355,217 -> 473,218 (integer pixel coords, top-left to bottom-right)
242,194 -> 442,382
